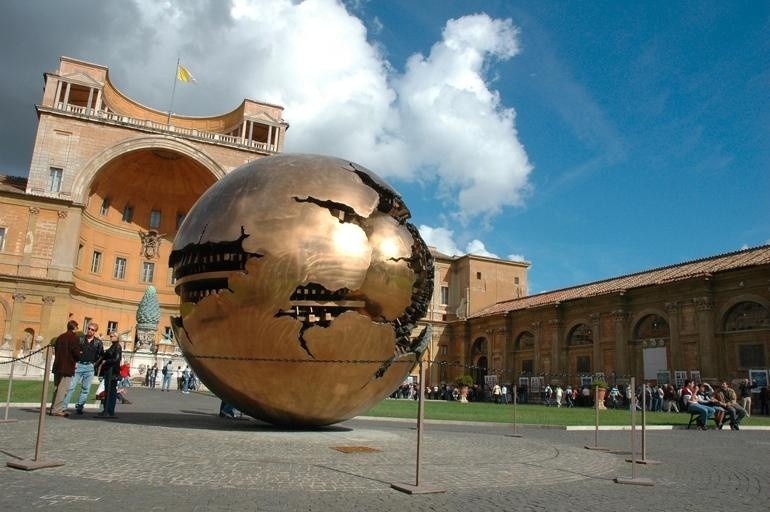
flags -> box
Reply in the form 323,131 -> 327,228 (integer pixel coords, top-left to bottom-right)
176,65 -> 197,84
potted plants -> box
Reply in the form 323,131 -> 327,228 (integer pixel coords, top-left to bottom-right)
455,375 -> 473,395
592,379 -> 610,400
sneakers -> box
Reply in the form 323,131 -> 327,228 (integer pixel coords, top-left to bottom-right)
222,410 -> 232,418
50,411 -> 68,416
123,398 -> 130,404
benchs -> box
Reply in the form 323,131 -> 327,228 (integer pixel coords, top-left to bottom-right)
678,398 -> 739,429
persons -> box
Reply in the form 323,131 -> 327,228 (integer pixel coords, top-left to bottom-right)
392,382 -> 420,400
426,383 -> 532,404
51,317 -> 199,416
545,383 -> 593,408
607,383 -> 683,414
218,399 -> 236,420
737,378 -> 768,417
680,379 -> 746,431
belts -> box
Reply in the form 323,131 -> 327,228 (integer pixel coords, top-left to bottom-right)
78,361 -> 92,365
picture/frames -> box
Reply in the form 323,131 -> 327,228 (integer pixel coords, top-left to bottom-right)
531,377 -> 539,392
580,376 -> 593,386
691,371 -> 701,386
748,369 -> 768,393
675,371 -> 687,388
538,377 -> 545,392
656,370 -> 671,385
519,378 -> 529,392
